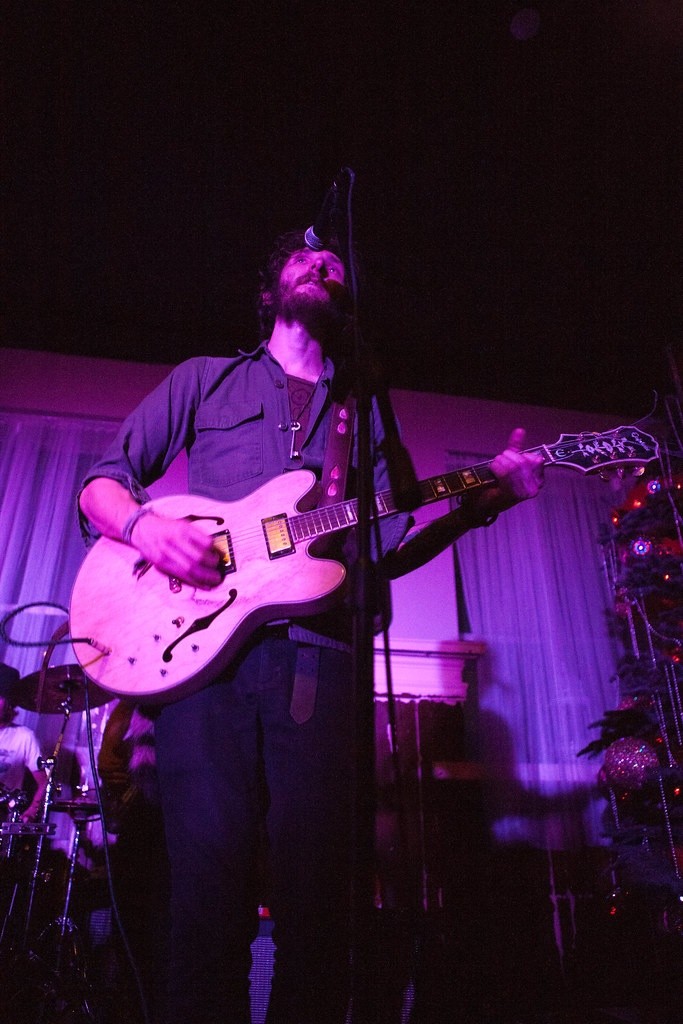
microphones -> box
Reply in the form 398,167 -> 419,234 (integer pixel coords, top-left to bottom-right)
304,166 -> 351,252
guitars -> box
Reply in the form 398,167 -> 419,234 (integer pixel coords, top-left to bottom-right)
64,420 -> 664,705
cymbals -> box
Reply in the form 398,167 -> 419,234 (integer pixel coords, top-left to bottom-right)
45,798 -> 103,822
13,662 -> 121,716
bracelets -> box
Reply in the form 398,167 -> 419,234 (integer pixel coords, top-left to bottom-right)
123,505 -> 154,547
456,491 -> 499,527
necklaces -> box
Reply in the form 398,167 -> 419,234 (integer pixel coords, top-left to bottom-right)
286,362 -> 327,458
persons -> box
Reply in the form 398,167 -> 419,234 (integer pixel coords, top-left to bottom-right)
98,695 -> 167,941
0,662 -> 49,826
74,220 -> 550,1024
